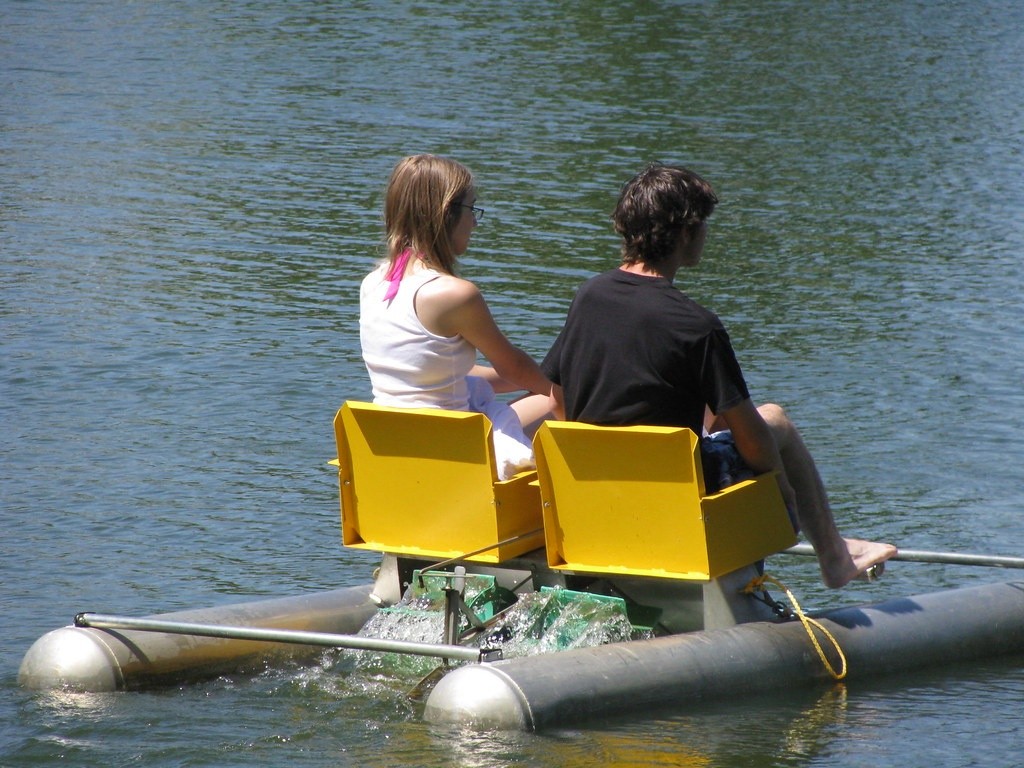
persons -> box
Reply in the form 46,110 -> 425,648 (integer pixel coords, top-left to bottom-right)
539,161 -> 898,588
358,155 -> 553,481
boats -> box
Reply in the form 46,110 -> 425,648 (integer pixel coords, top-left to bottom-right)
15,401 -> 1021,738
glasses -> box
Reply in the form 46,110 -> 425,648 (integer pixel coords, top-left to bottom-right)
451,201 -> 484,220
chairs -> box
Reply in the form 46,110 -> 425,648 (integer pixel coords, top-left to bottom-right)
326,401 -> 800,634
